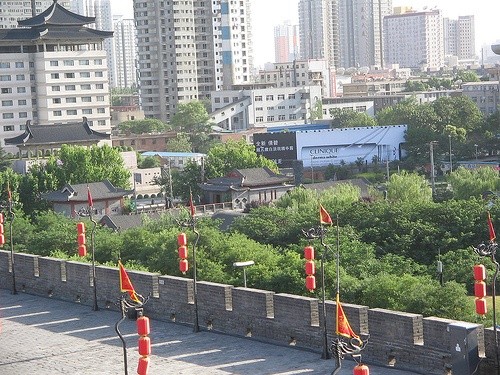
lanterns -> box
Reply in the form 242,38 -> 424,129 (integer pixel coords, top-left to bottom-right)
0,223 -> 4,234
0,234 -> 4,247
136,316 -> 150,336
475,298 -> 487,319
78,233 -> 85,245
474,281 -> 487,298
304,246 -> 314,261
474,263 -> 485,281
305,260 -> 315,275
352,363 -> 369,375
180,259 -> 188,275
138,336 -> 151,356
179,245 -> 187,259
178,233 -> 187,245
306,275 -> 316,293
137,357 -> 151,375
0,213 -> 3,223
77,222 -> 84,233
78,245 -> 86,257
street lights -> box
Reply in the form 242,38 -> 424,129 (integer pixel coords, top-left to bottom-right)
176,218 -> 200,333
0,199 -> 18,295
470,241 -> 500,375
114,291 -> 153,375
329,332 -> 372,375
301,226 -> 331,360
73,205 -> 100,311
